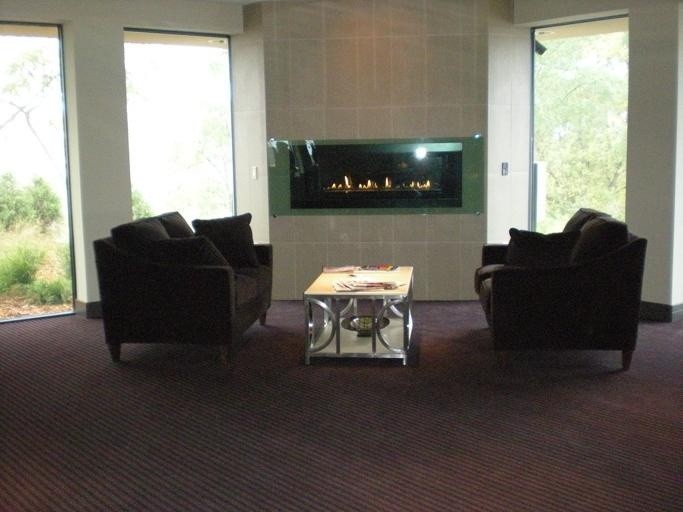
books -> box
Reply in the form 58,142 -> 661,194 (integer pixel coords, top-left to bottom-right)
323,264 -> 400,292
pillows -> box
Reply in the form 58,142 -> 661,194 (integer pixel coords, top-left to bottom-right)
564,210 -> 610,231
192,213 -> 258,266
504,229 -> 580,266
572,218 -> 628,262
150,237 -> 229,265
111,218 -> 169,263
159,212 -> 193,237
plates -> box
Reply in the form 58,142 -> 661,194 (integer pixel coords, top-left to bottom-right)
341,315 -> 390,334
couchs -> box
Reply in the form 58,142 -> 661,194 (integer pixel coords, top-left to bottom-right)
93,212 -> 273,362
474,208 -> 647,370
